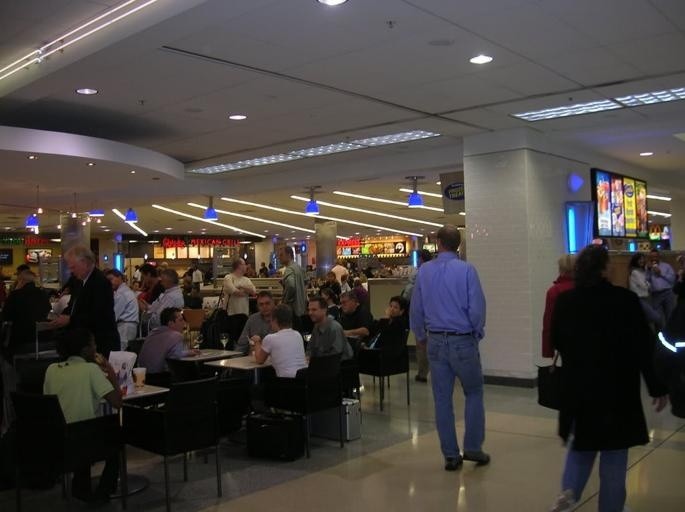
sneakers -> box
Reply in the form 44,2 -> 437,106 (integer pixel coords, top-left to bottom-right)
445,455 -> 463,470
416,375 -> 427,382
551,495 -> 571,512
462,450 -> 490,463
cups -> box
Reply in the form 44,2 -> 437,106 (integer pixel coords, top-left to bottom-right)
132,367 -> 146,389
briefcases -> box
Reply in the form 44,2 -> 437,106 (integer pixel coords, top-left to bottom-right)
312,397 -> 362,442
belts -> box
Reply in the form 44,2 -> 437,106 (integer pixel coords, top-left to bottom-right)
430,330 -> 468,337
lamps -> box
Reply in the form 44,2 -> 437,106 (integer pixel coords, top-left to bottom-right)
403,174 -> 430,210
299,183 -> 321,216
204,195 -> 219,223
123,205 -> 139,224
24,205 -> 39,229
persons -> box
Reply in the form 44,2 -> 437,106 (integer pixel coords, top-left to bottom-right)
409,223 -> 490,472
539,247 -> 684,447
548,244 -> 669,512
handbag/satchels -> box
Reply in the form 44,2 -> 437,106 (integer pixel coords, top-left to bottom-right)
208,308 -> 229,332
108,351 -> 137,394
537,352 -> 562,412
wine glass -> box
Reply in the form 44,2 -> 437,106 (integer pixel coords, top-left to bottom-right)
220,332 -> 229,351
193,333 -> 203,351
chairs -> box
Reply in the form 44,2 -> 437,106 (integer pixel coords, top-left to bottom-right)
204,283 -> 413,460
0,283 -> 219,510
